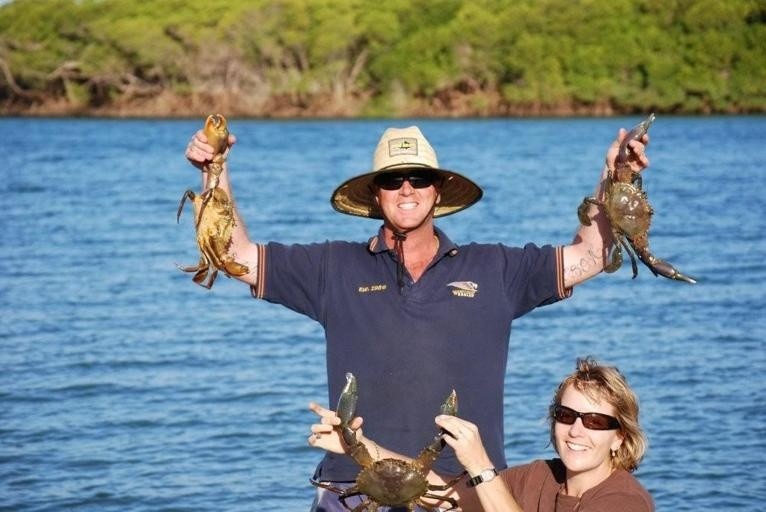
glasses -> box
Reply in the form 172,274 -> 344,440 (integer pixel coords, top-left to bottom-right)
372,170 -> 440,190
553,404 -> 620,430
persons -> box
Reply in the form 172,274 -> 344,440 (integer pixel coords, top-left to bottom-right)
306,356 -> 655,512
183,125 -> 649,511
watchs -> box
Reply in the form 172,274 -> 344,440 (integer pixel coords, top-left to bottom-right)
465,467 -> 500,488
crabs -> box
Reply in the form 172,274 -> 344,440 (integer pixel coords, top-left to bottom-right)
308,371 -> 468,512
176,113 -> 250,289
577,112 -> 698,285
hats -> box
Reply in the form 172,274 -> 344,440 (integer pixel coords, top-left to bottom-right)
330,126 -> 483,219
